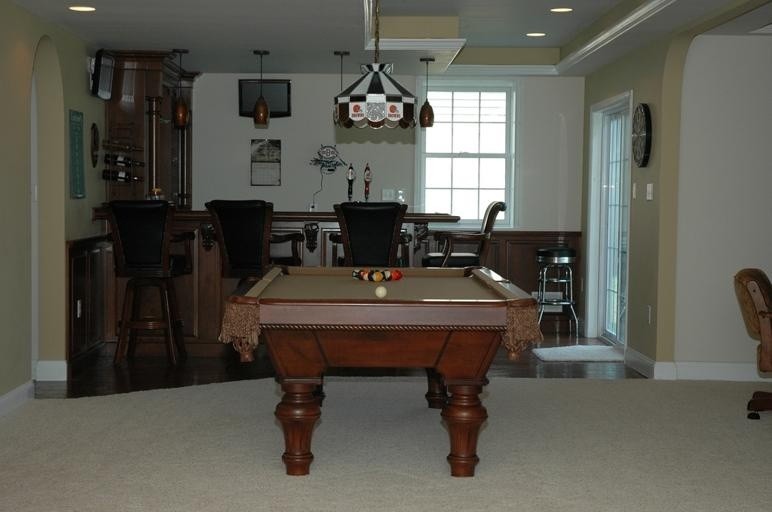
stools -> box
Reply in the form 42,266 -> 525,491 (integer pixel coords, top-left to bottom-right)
531,243 -> 584,349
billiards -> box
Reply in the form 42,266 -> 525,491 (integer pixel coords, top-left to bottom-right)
375,286 -> 387,298
352,269 -> 404,282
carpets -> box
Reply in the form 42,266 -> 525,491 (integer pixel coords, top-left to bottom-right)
2,359 -> 771,512
528,341 -> 626,366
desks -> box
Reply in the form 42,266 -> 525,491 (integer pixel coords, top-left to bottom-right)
89,200 -> 461,359
214,258 -> 548,479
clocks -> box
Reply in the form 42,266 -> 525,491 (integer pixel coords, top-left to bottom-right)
628,103 -> 653,170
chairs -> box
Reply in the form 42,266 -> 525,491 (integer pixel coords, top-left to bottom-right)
97,199 -> 199,367
328,200 -> 414,268
420,201 -> 508,268
733,267 -> 771,423
204,198 -> 305,291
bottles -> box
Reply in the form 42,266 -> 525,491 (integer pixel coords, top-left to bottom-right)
101,140 -> 146,183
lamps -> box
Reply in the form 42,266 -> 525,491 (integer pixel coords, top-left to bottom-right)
418,58 -> 439,129
170,47 -> 194,131
249,48 -> 273,126
330,0 -> 418,132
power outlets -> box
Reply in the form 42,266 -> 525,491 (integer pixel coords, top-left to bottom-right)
308,203 -> 318,213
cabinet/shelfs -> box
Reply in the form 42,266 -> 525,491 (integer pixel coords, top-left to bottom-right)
102,46 -> 201,213
